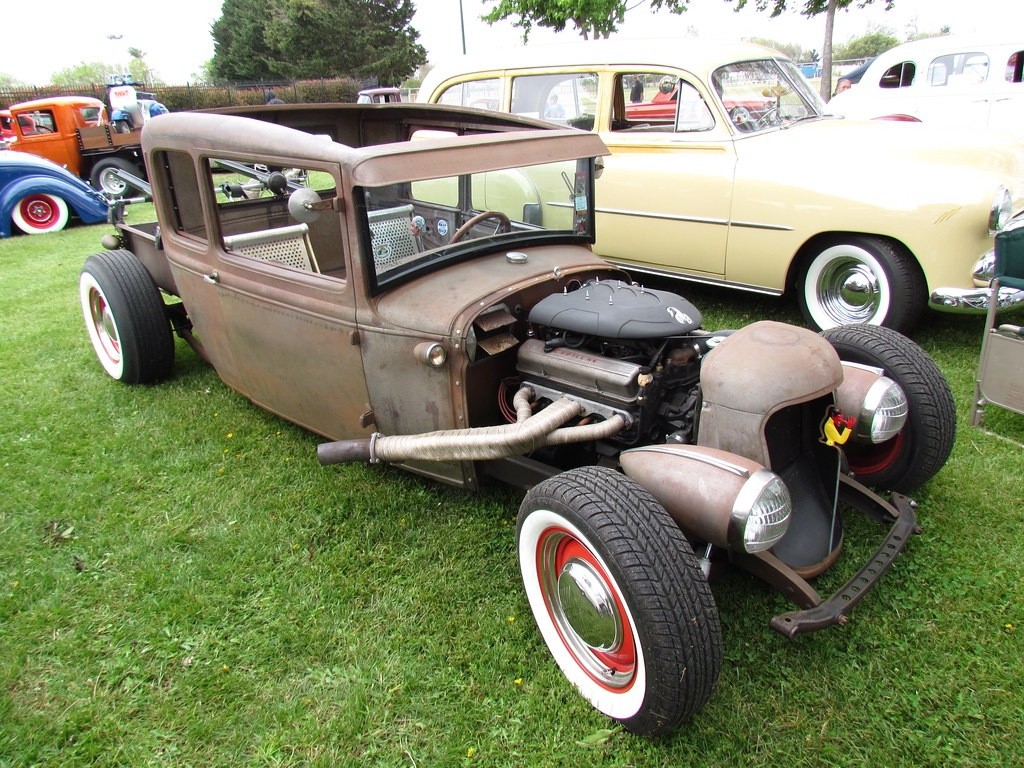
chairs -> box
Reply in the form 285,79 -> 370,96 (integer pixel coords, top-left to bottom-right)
967,228 -> 1024,449
368,205 -> 419,269
223,222 -> 321,273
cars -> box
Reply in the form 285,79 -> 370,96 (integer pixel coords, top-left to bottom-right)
0,94 -> 172,239
625,32 -> 1023,233
77,98 -> 962,742
392,40 -> 1024,340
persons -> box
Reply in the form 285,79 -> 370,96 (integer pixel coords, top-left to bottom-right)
545,94 -> 565,118
629,75 -> 644,104
831,78 -> 851,99
265,92 -> 284,106
